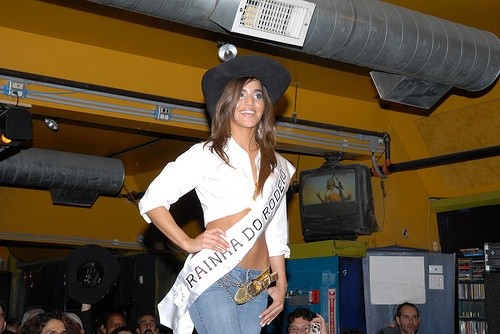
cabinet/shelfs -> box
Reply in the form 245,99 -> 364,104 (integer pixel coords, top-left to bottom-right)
456,254 -> 489,334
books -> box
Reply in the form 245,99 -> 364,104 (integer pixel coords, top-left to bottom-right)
458,302 -> 488,334
458,284 -> 485,300
456,248 -> 485,278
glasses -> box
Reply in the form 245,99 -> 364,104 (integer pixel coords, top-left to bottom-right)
289,325 -> 310,334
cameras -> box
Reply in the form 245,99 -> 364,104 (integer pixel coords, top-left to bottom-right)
310,323 -> 321,334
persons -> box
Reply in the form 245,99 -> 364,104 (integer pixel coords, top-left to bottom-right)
374,301 -> 421,334
138,55 -> 296,334
0,303 -> 159,334
286,307 -> 328,334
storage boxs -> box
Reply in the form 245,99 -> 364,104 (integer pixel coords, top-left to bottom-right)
469,301 -> 486,318
457,300 -> 470,318
485,244 -> 500,272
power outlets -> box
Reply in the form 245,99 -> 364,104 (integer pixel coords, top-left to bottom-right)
8,80 -> 26,99
402,228 -> 408,239
157,105 -> 172,122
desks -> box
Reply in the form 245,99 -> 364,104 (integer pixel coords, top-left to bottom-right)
482,270 -> 500,334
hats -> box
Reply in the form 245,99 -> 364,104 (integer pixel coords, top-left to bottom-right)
327,180 -> 335,188
201,55 -> 292,120
63,312 -> 83,330
21,308 -> 46,326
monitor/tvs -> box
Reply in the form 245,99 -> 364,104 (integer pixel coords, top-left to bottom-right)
300,164 -> 376,243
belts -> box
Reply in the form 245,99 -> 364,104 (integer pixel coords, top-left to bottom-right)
234,264 -> 279,304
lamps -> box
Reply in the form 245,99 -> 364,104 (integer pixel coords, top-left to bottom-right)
44,117 -> 61,131
217,41 -> 239,62
